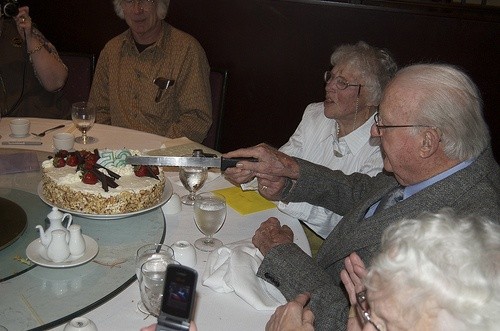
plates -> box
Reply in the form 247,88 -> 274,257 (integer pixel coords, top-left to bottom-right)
37,177 -> 174,219
26,233 -> 99,267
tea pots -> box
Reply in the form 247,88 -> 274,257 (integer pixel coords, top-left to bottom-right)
36,206 -> 73,248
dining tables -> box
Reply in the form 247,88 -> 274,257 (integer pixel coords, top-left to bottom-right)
0,117 -> 312,331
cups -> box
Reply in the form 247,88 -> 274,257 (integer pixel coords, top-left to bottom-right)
160,194 -> 182,214
140,257 -> 181,318
10,119 -> 30,137
64,317 -> 98,331
168,241 -> 197,268
52,133 -> 74,151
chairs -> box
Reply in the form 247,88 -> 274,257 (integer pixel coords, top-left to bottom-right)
202,68 -> 230,151
55,53 -> 96,120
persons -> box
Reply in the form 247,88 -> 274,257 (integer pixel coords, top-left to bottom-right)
224,40 -> 397,239
222,64 -> 499,331
265,206 -> 500,331
0,0 -> 76,120
87,0 -> 213,145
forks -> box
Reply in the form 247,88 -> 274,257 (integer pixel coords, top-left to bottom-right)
31,125 -> 66,136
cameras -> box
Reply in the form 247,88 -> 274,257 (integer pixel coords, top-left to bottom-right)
0,0 -> 22,17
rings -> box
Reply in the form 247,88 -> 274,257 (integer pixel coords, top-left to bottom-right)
262,185 -> 268,190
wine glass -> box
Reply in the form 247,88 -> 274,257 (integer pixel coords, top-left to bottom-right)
180,167 -> 208,204
193,192 -> 227,253
71,101 -> 97,144
134,244 -> 174,315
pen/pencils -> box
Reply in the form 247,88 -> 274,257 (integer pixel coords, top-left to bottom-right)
2,142 -> 42,145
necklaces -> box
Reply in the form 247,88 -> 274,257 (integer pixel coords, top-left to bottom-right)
336,107 -> 370,137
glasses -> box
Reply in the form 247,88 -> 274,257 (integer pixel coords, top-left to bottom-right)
374,113 -> 441,142
324,70 -> 374,90
356,289 -> 381,331
121,0 -> 159,8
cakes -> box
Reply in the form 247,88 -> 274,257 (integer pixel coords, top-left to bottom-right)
41,148 -> 165,215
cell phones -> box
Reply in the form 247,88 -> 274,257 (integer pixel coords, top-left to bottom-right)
156,263 -> 198,331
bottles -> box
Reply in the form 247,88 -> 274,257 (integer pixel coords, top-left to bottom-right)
48,223 -> 85,263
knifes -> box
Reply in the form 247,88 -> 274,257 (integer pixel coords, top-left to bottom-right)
126,156 -> 258,171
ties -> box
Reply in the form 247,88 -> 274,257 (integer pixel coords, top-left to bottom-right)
374,185 -> 404,214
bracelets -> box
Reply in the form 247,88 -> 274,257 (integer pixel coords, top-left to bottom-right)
29,45 -> 45,55
